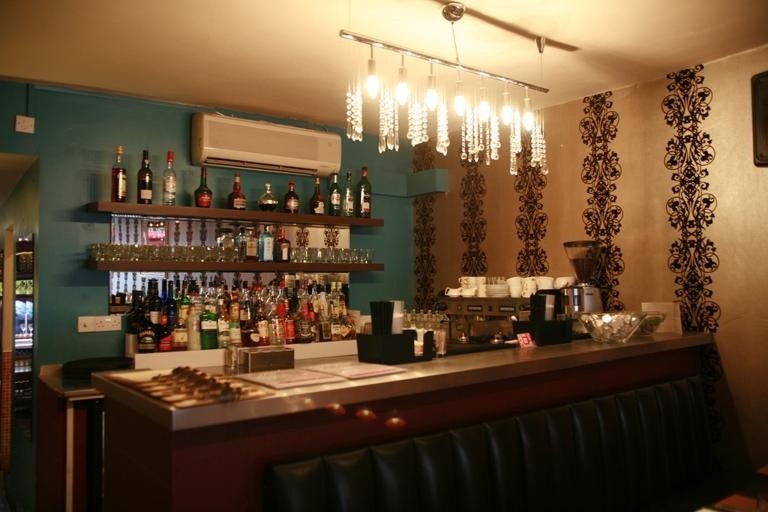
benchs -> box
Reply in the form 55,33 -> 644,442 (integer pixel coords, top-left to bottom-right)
261,372 -> 726,512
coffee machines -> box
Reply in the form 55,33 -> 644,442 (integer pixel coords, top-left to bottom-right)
560,242 -> 609,314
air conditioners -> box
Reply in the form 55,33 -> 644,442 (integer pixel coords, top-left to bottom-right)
189,111 -> 343,179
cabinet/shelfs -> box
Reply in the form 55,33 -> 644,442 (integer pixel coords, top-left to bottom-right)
80,200 -> 385,273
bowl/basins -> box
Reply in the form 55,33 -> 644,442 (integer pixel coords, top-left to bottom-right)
635,310 -> 667,336
576,309 -> 648,344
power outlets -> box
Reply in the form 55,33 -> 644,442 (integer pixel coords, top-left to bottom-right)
95,315 -> 122,332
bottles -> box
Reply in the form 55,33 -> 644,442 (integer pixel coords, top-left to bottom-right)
402,308 -> 448,356
123,274 -> 355,351
109,141 -> 373,218
237,222 -> 291,263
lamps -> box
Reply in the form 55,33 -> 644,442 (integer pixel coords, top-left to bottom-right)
334,1 -> 551,178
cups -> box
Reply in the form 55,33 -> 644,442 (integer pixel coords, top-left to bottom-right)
444,275 -> 575,299
290,247 -> 374,263
87,239 -> 234,262
